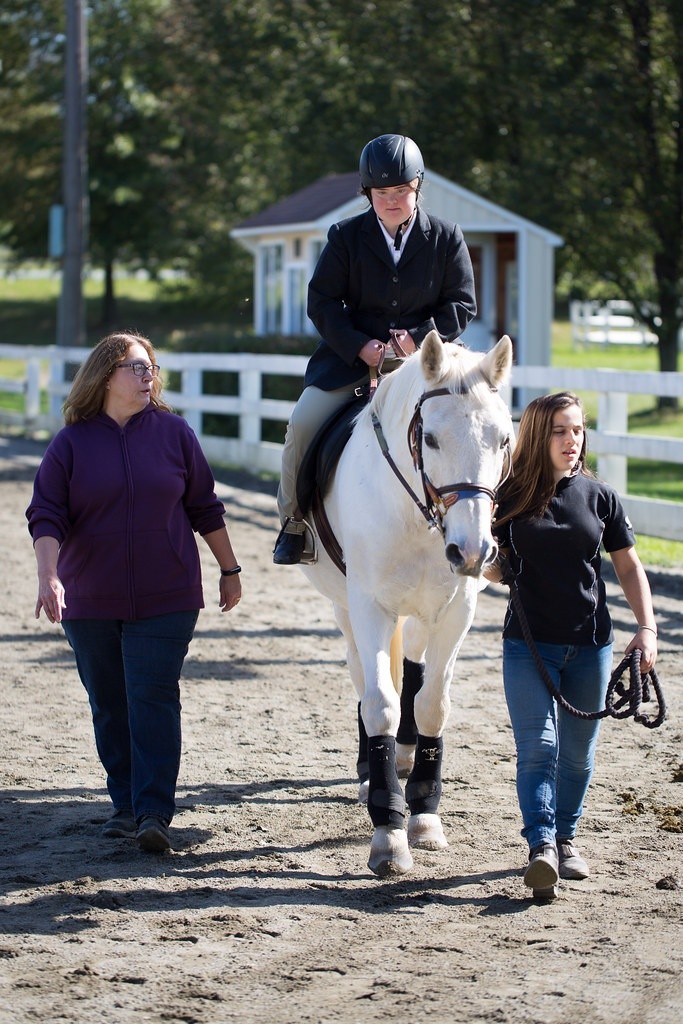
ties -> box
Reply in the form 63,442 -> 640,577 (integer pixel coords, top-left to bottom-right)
392,243 -> 402,265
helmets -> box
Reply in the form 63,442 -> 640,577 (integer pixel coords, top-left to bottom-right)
359,134 -> 425,188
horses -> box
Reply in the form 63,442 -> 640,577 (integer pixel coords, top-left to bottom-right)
282,315 -> 522,878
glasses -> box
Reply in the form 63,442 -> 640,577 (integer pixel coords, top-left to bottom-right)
116,361 -> 162,378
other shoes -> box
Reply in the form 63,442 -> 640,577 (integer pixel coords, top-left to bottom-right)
103,809 -> 136,838
273,531 -> 305,565
137,815 -> 171,852
558,838 -> 590,878
524,843 -> 559,898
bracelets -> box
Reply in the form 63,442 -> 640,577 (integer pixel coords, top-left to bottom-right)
221,565 -> 242,576
638,626 -> 658,639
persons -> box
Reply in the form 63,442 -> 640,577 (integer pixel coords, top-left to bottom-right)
476,389 -> 659,905
28,334 -> 242,856
271,131 -> 478,569
